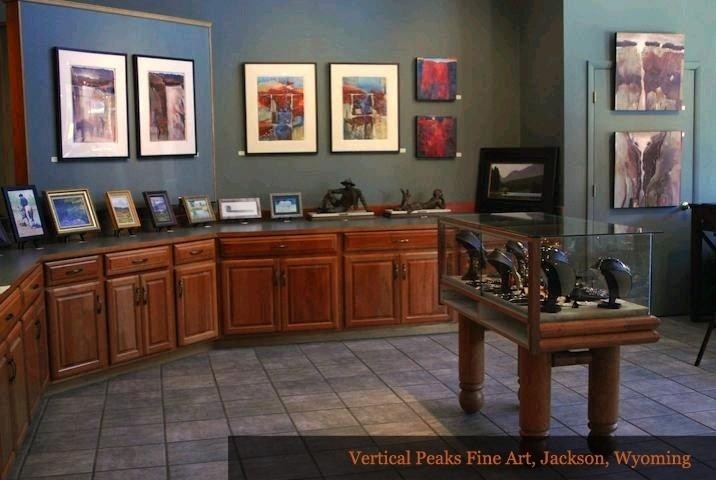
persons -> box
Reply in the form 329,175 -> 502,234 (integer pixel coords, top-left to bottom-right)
19,194 -> 28,219
399,188 -> 446,210
315,178 -> 373,213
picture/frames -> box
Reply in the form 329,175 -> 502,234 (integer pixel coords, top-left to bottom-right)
328,62 -> 400,153
54,47 -> 130,160
134,55 -> 199,156
475,148 -> 558,214
3,185 -> 302,244
244,62 -> 318,155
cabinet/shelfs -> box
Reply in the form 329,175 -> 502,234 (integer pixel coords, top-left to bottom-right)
0,226 -> 561,477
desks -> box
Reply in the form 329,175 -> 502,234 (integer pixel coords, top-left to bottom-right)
689,203 -> 716,322
